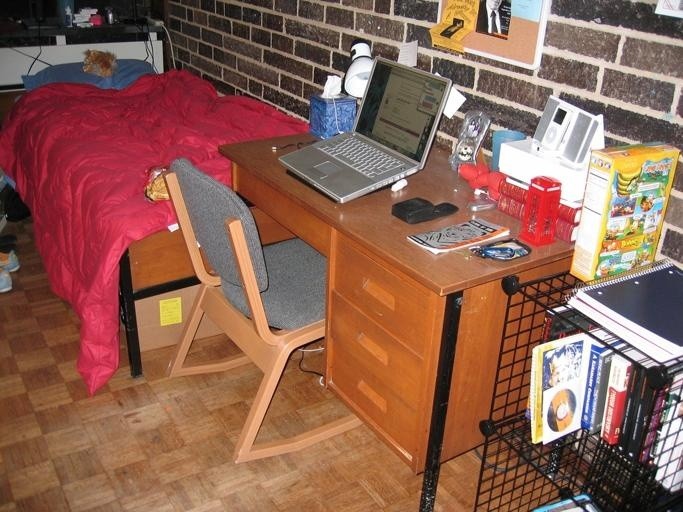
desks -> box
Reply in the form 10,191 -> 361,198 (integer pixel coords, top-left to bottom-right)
1,14 -> 166,92
213,123 -> 581,481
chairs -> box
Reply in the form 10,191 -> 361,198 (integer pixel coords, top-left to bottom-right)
157,152 -> 367,469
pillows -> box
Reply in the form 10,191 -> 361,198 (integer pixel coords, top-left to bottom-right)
19,55 -> 159,93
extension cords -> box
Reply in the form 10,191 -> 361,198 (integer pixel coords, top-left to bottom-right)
145,14 -> 164,26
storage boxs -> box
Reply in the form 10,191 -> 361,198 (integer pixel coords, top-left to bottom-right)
131,280 -> 227,355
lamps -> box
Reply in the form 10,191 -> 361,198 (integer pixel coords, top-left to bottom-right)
335,35 -> 374,101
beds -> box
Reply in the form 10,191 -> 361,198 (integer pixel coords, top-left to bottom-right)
0,66 -> 311,381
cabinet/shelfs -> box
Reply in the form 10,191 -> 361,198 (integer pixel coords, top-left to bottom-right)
472,248 -> 683,510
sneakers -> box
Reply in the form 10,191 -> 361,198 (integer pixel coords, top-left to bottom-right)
0,248 -> 20,293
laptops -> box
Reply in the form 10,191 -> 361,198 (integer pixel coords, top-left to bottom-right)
277,58 -> 453,204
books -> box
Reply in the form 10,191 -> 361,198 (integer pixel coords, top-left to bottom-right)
529,263 -> 683,497
569,141 -> 680,284
406,216 -> 510,255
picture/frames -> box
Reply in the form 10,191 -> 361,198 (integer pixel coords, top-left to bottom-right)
434,0 -> 555,74
445,108 -> 491,173
